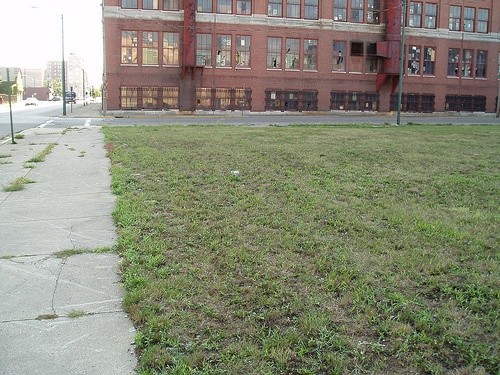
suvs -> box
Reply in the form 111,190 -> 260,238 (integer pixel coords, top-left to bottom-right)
64,92 -> 76,104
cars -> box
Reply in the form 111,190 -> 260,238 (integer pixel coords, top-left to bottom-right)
25,97 -> 39,106
47,96 -> 60,101
